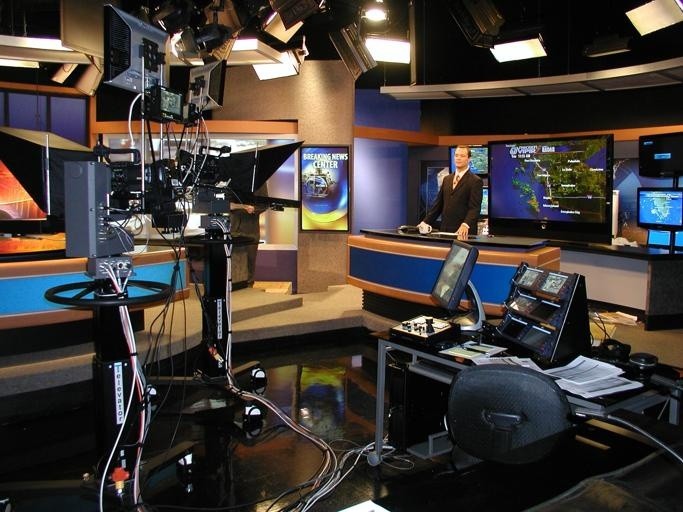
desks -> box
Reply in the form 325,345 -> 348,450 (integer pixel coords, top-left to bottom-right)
348,229 -> 560,323
370,321 -> 683,463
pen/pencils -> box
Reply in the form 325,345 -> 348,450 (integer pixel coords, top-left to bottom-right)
464,347 -> 490,355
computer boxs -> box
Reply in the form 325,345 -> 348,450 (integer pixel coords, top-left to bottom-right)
386,363 -> 441,452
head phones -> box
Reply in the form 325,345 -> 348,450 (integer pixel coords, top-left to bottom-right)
596,338 -> 660,383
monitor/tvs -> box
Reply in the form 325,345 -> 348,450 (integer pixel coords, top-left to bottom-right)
407,132 -> 683,252
0,5 -> 353,272
431,239 -> 488,331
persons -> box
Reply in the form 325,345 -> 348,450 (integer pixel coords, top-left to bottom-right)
416,144 -> 483,236
225,180 -> 268,287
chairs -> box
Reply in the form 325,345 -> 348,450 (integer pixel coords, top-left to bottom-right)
448,364 -> 574,471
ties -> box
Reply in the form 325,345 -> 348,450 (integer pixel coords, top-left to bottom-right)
452,175 -> 460,190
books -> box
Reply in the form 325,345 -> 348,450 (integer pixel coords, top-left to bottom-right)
446,340 -> 493,358
